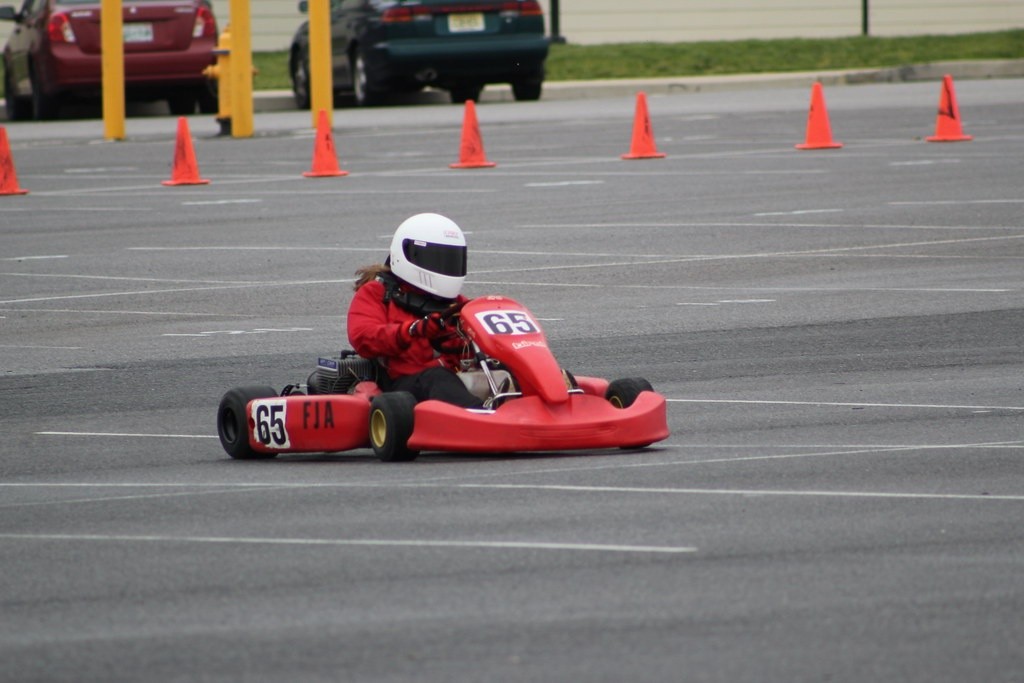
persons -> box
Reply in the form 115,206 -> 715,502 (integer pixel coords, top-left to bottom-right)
347,213 -> 579,410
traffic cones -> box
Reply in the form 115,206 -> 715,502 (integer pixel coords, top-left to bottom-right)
300,107 -> 348,177
159,116 -> 210,186
0,124 -> 29,195
796,81 -> 845,148
618,92 -> 666,158
449,99 -> 496,169
924,75 -> 973,141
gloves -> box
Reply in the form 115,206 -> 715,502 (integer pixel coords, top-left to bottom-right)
408,311 -> 455,339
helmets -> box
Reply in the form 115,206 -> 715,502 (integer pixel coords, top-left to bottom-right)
389,213 -> 468,299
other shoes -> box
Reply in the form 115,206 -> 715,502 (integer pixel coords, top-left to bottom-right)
483,377 -> 522,411
562,369 -> 578,391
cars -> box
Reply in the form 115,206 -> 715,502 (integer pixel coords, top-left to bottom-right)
0,0 -> 222,121
288,0 -> 550,111
